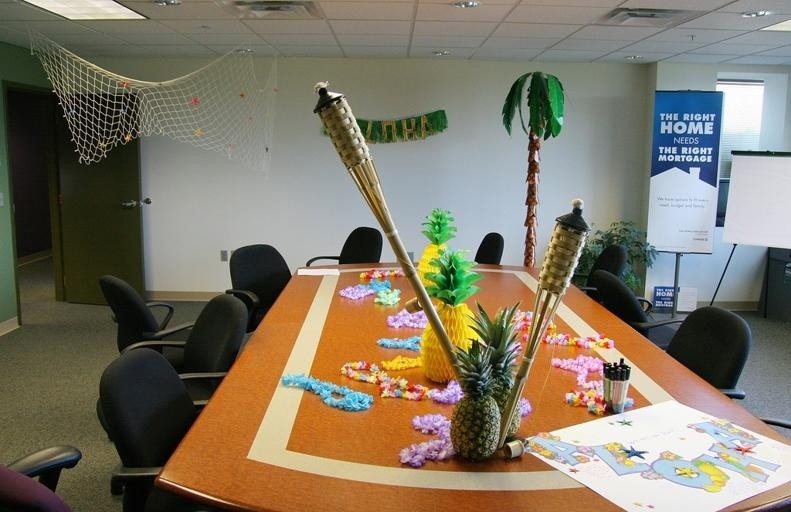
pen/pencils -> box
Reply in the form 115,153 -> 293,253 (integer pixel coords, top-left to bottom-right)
603,358 -> 631,380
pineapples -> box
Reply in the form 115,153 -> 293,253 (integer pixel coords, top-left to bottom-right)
449,338 -> 500,464
465,300 -> 525,440
417,207 -> 457,288
418,248 -> 481,384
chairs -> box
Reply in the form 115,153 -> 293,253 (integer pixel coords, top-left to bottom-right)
101,274 -> 198,367
666,305 -> 752,400
99,350 -> 209,512
474,232 -> 504,265
0,445 -> 83,510
306,226 -> 382,266
571,244 -> 626,302
226,243 -> 293,334
594,268 -> 685,350
98,296 -> 248,443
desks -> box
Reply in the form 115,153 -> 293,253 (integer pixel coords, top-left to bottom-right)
153,262 -> 791,512
